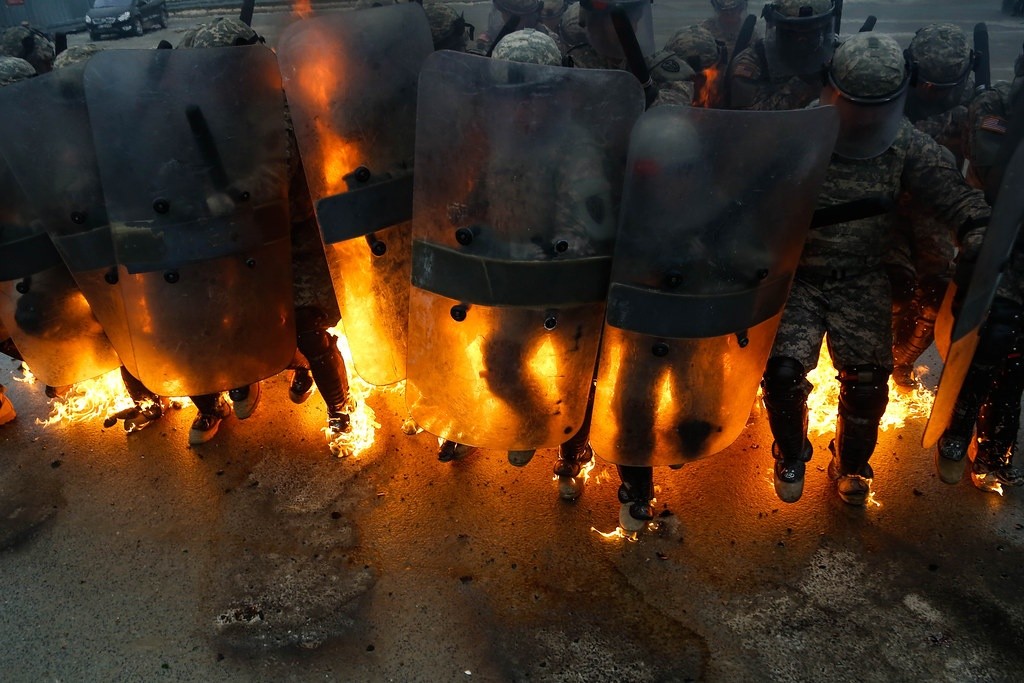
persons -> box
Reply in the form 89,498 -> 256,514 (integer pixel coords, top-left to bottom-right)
1,0 -> 1024,541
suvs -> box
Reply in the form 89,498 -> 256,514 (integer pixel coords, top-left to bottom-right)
86,0 -> 169,40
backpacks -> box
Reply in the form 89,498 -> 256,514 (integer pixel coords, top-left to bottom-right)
0,26 -> 56,72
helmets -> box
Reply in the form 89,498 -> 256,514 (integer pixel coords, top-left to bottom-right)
354,0 -> 395,10
0,57 -> 36,85
663,24 -> 722,71
909,22 -> 972,86
486,29 -> 562,92
54,44 -> 105,68
763,0 -> 836,27
826,31 -> 909,103
411,0 -> 463,50
169,17 -> 261,84
490,0 -> 592,45
711,0 -> 749,13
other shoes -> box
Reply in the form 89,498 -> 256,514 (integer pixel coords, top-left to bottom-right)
508,416 -> 550,466
827,450 -> 873,505
289,361 -> 318,404
402,391 -> 437,435
0,387 -> 16,427
45,385 -> 75,397
554,443 -> 593,502
327,404 -> 356,457
229,380 -> 262,420
937,432 -> 966,485
968,434 -> 1023,488
772,437 -> 813,503
438,419 -> 479,462
188,398 -> 232,444
619,480 -> 654,531
